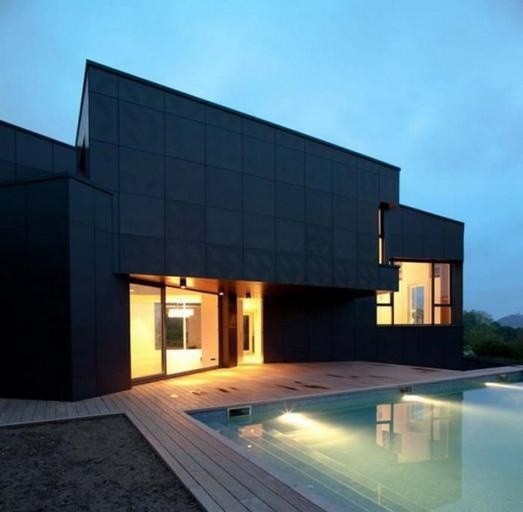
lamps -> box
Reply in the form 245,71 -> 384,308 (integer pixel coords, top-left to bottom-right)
180,278 -> 186,289
